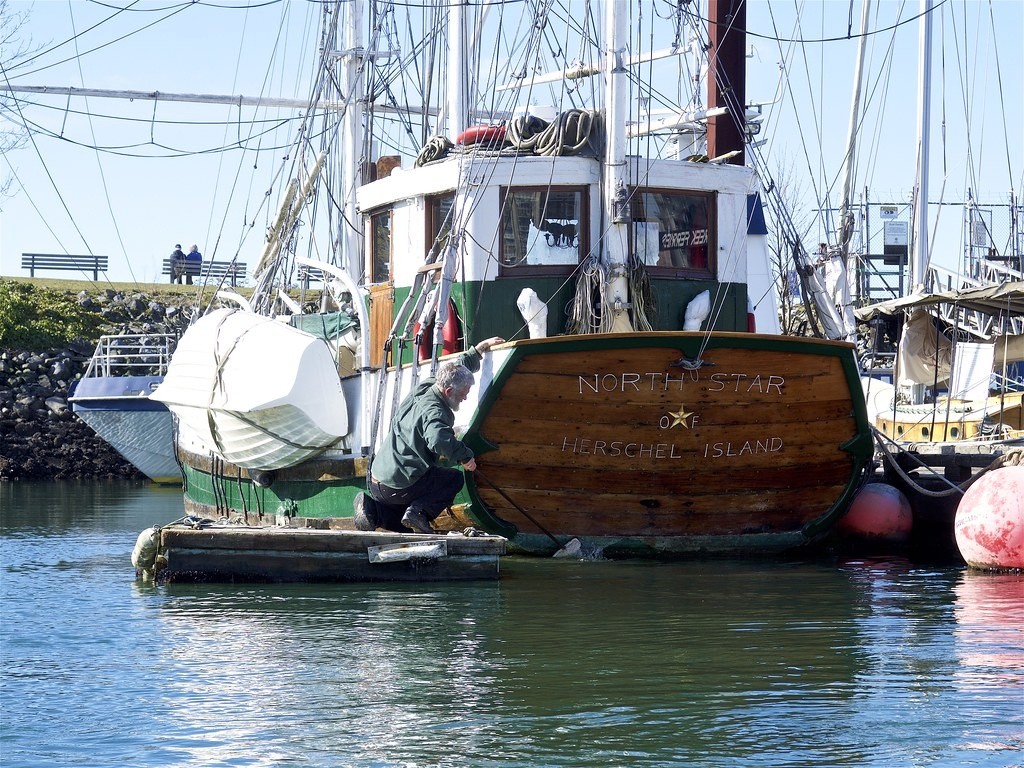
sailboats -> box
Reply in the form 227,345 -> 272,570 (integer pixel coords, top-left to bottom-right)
0,3 -> 1022,587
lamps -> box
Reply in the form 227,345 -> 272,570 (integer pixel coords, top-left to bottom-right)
539,198 -> 579,249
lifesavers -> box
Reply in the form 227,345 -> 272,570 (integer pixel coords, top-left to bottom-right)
412,294 -> 459,364
456,123 -> 506,148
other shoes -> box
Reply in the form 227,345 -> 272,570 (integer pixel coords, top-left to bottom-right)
402,505 -> 434,533
352,491 -> 375,532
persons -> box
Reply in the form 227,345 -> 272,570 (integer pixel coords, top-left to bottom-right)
351,333 -> 510,536
168,244 -> 186,284
184,244 -> 203,286
811,243 -> 832,271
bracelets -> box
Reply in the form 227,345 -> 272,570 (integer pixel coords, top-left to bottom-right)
474,344 -> 485,351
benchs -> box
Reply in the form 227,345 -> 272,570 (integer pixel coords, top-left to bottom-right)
162,258 -> 247,288
297,263 -> 346,289
21,252 -> 108,281
